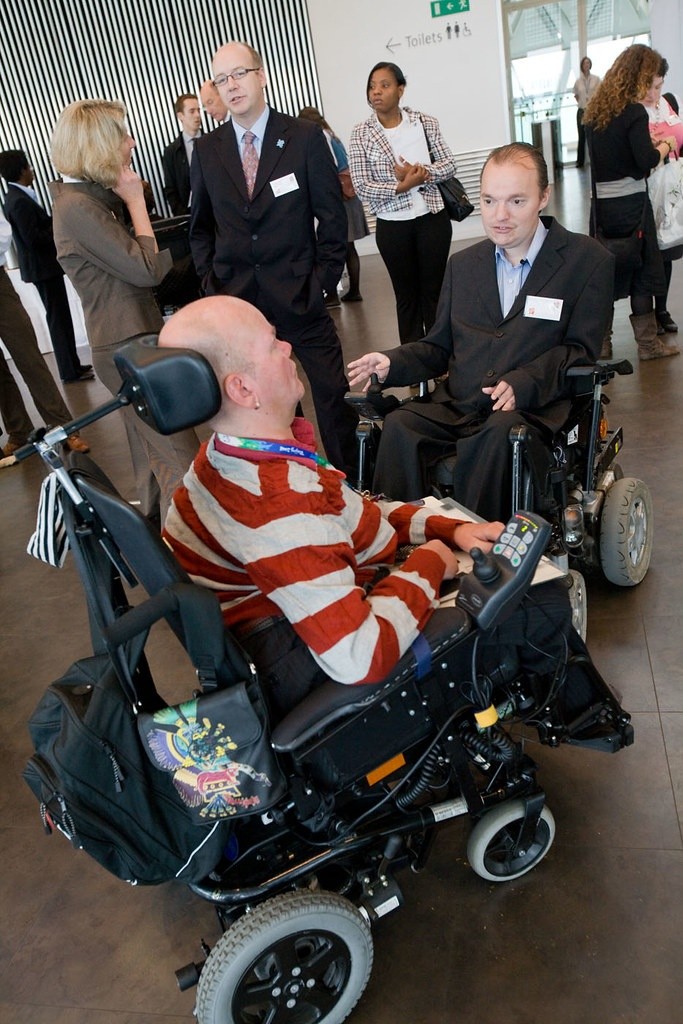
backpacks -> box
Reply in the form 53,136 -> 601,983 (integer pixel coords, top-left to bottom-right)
21,453 -> 227,892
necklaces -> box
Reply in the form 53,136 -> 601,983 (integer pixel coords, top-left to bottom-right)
584,78 -> 591,101
650,103 -> 660,133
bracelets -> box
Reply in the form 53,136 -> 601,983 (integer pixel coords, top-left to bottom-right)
659,140 -> 672,156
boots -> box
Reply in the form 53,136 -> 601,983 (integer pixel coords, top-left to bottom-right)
600,320 -> 615,359
628,311 -> 681,360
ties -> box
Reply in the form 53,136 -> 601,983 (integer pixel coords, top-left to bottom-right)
241,130 -> 259,199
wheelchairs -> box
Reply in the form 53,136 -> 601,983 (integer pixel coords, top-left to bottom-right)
2,346 -> 634,1023
343,330 -> 652,590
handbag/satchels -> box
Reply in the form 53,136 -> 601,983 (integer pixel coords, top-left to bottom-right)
595,237 -> 643,272
339,167 -> 355,199
435,174 -> 476,222
134,680 -> 290,826
648,142 -> 683,251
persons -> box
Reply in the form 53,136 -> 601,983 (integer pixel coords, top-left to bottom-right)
159,296 -> 622,738
0,216 -> 90,458
47,100 -> 201,534
573,44 -> 683,360
350,63 -> 457,395
0,149 -> 95,384
348,141 -> 617,525
188,43 -> 371,488
163,81 -> 370,306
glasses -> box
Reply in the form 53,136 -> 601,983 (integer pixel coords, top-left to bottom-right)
211,67 -> 258,88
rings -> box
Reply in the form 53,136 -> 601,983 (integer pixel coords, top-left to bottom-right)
124,167 -> 131,171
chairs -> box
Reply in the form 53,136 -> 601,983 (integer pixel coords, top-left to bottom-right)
127,213 -> 200,311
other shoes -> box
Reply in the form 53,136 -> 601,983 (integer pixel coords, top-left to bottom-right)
2,441 -> 23,457
324,295 -> 341,311
655,310 -> 679,336
340,287 -> 363,302
63,364 -> 95,384
66,435 -> 90,453
